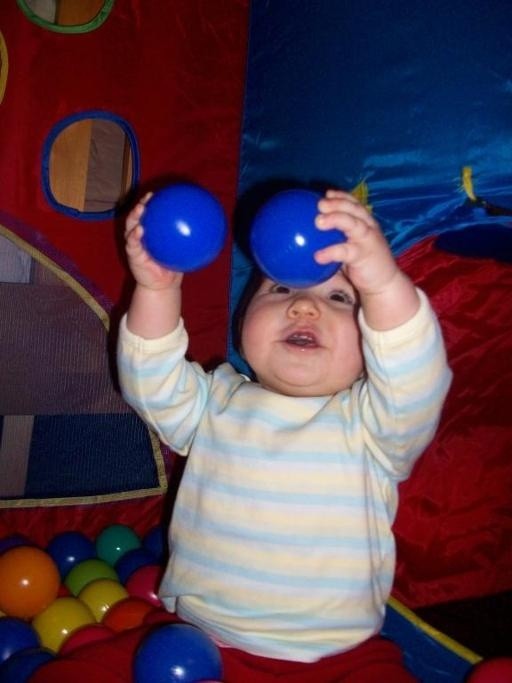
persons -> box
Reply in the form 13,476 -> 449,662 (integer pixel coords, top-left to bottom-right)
36,193 -> 454,681
